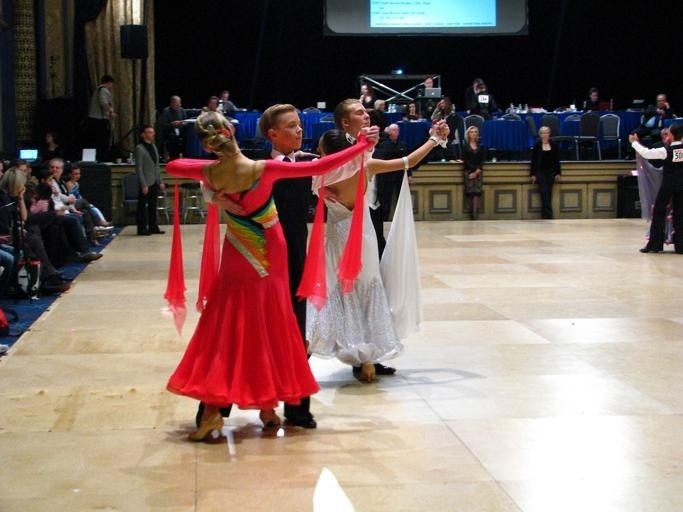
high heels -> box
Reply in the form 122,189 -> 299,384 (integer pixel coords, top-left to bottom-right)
189,411 -> 224,441
260,410 -> 281,430
359,362 -> 375,384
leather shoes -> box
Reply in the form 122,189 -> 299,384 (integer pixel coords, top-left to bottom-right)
640,245 -> 659,253
289,419 -> 316,428
149,229 -> 165,234
76,254 -> 94,263
138,231 -> 151,235
84,254 -> 101,260
352,363 -> 396,375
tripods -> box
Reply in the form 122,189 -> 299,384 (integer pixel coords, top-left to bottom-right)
115,60 -> 141,148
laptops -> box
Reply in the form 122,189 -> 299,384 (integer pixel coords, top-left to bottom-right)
425,88 -> 441,98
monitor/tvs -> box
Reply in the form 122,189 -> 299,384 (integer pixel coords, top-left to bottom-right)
17,148 -> 38,163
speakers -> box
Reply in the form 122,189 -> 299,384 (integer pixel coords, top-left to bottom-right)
618,175 -> 641,218
120,25 -> 148,59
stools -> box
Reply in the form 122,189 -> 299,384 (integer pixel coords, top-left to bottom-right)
182,183 -> 205,225
155,182 -> 170,225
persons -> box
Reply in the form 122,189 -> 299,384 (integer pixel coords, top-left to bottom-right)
163,110 -> 374,441
162,89 -> 244,167
529,126 -> 561,219
584,88 -> 600,113
135,124 -> 166,236
628,94 -> 683,255
305,123 -> 444,383
0,132 -> 117,355
196,103 -> 380,429
357,75 -> 500,222
88,76 -> 118,160
312,99 -> 451,375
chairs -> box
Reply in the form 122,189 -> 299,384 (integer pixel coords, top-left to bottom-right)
120,173 -> 149,225
